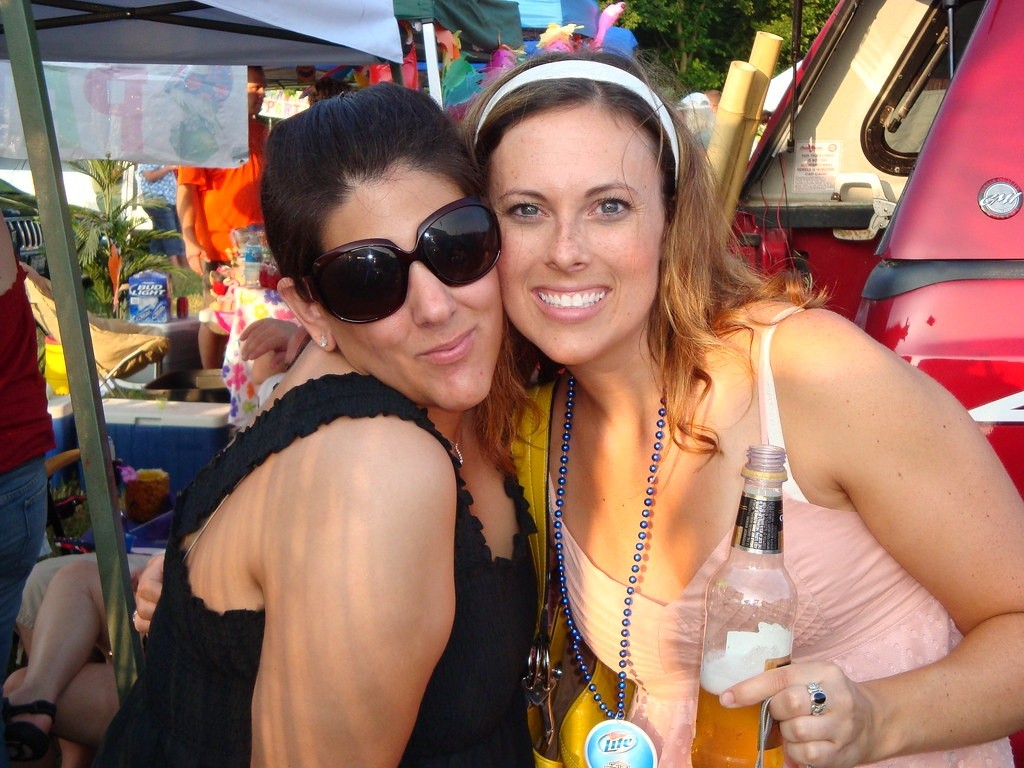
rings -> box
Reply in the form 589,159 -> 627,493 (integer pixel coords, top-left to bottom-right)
133,610 -> 137,622
808,683 -> 828,716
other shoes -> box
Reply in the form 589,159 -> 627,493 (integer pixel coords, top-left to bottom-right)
0,697 -> 63,767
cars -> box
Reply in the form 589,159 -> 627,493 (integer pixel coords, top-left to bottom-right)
0,178 -> 109,284
722,0 -> 1024,502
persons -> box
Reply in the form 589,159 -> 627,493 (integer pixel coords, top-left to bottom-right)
1,52 -> 1024,768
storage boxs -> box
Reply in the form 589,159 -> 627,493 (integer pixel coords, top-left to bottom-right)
77,398 -> 234,556
115,316 -> 200,392
130,268 -> 173,324
47,384 -> 103,488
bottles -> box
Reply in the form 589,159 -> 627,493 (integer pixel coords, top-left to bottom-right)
243,225 -> 262,288
691,444 -> 797,768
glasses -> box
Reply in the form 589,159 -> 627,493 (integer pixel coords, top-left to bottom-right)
295,195 -> 502,323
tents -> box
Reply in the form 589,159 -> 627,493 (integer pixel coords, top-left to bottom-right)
1,0 -> 597,704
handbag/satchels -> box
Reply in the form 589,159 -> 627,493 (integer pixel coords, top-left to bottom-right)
506,379 -> 635,768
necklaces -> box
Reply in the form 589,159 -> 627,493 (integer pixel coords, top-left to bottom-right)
553,359 -> 668,768
446,435 -> 464,465
